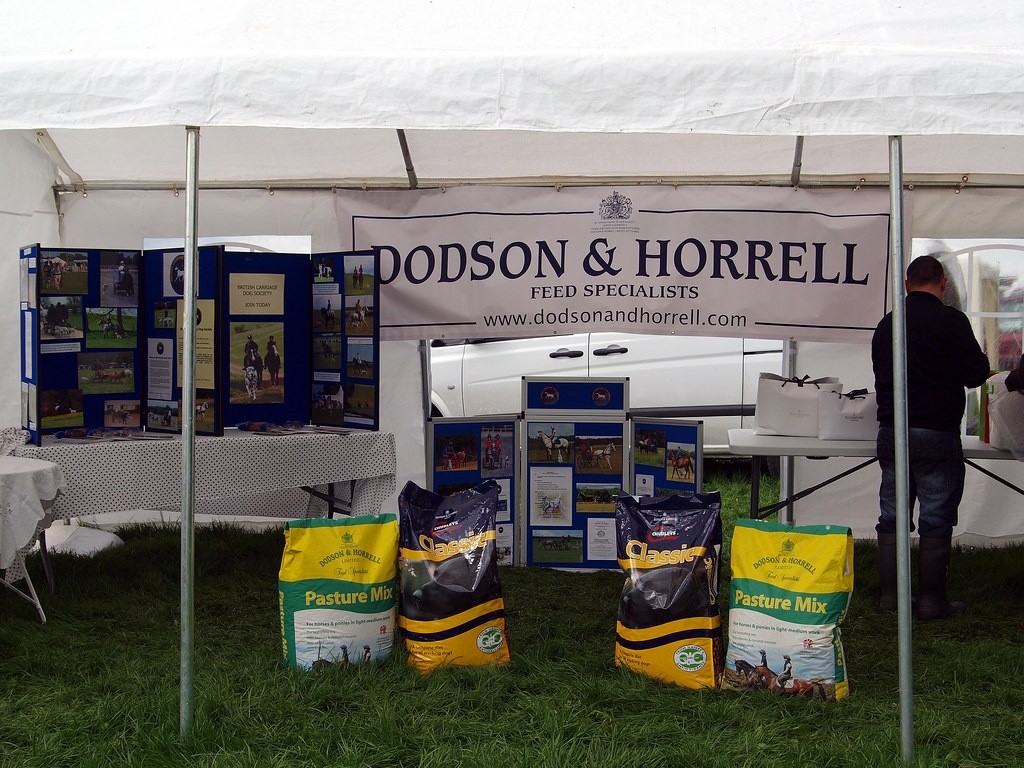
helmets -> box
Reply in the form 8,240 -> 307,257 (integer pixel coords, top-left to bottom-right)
247,334 -> 253,339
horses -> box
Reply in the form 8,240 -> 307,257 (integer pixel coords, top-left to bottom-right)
536,430 -> 571,462
244,341 -> 281,388
123,266 -> 134,295
735,658 -> 826,701
348,304 -> 369,328
352,357 -> 368,376
196,401 -> 209,419
96,368 -> 132,383
320,307 -> 336,330
577,437 -> 694,505
97,319 -> 117,339
352,272 -> 363,290
487,443 -> 497,472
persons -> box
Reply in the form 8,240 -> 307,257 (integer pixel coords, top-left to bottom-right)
756,649 -> 767,686
561,535 -> 571,548
587,445 -> 593,452
51,400 -> 69,416
116,260 -> 134,297
676,446 -> 682,461
777,655 -> 792,687
43,257 -> 61,290
242,335 -> 279,370
339,645 -> 348,662
640,434 -> 656,454
148,405 -> 171,427
317,264 -> 372,426
106,314 -> 111,325
363,645 -> 371,663
114,405 -> 127,425
442,442 -> 465,470
163,302 -> 168,318
871,255 -> 989,621
549,425 -> 557,444
485,434 -> 501,469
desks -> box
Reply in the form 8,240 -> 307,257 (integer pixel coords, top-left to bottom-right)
0,427 -> 397,595
0,455 -> 67,625
727,428 -> 1024,520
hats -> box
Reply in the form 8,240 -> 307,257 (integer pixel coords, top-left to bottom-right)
449,442 -> 453,445
269,335 -> 274,339
120,260 -> 124,264
494,434 -> 500,439
328,300 -> 330,302
486,433 -> 492,438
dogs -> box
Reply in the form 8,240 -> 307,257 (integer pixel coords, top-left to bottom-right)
159,316 -> 174,327
504,455 -> 510,469
54,326 -> 76,337
115,332 -> 121,339
102,284 -> 109,294
241,365 -> 258,401
81,377 -> 87,383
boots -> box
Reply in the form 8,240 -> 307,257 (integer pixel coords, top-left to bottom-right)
916,533 -> 967,620
877,531 -> 897,610
